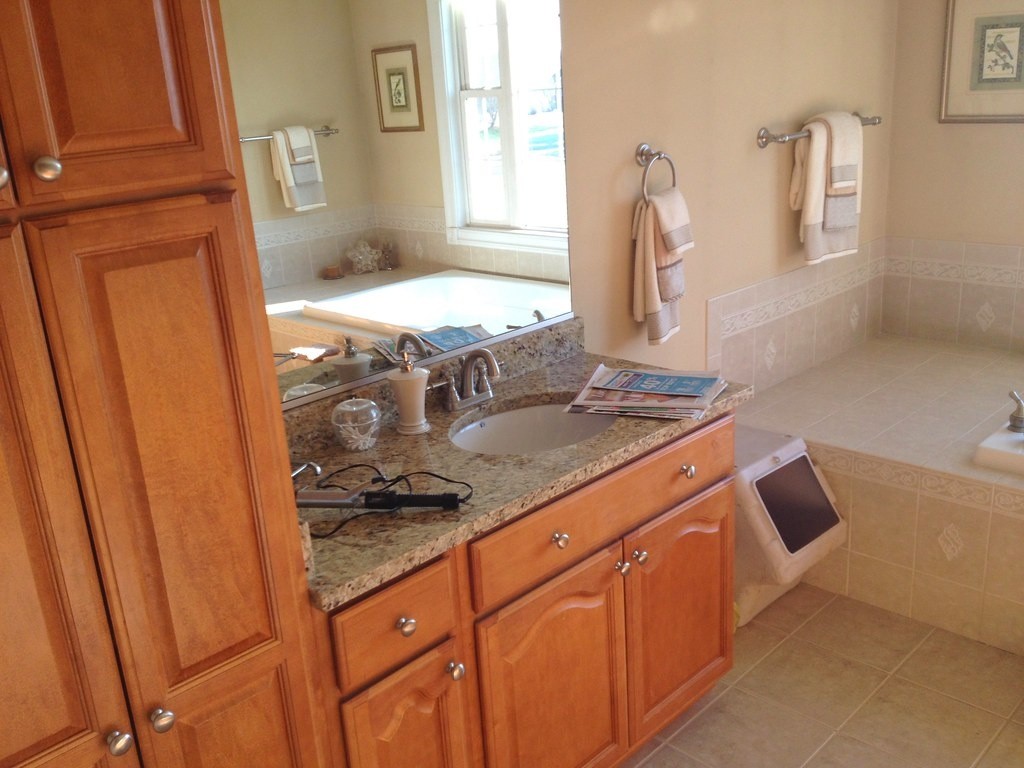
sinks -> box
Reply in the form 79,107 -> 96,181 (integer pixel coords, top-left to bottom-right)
447,390 -> 619,454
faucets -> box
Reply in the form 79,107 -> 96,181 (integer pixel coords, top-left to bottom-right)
396,332 -> 429,360
460,347 -> 499,396
531,307 -> 546,324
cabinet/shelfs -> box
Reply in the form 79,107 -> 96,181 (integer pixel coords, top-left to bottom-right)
466,406 -> 738,767
311,550 -> 472,768
2,188 -> 339,767
1,0 -> 247,216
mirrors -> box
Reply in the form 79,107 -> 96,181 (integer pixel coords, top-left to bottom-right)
220,1 -> 574,411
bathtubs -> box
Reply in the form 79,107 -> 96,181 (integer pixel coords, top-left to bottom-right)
312,267 -> 572,337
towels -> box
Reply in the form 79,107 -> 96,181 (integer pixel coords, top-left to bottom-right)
269,125 -> 328,212
630,186 -> 694,345
788,111 -> 864,265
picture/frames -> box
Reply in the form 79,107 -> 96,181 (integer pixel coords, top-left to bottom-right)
371,43 -> 426,133
938,0 -> 1024,124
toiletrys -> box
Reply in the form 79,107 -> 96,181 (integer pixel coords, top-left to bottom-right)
387,350 -> 431,435
331,333 -> 374,383
